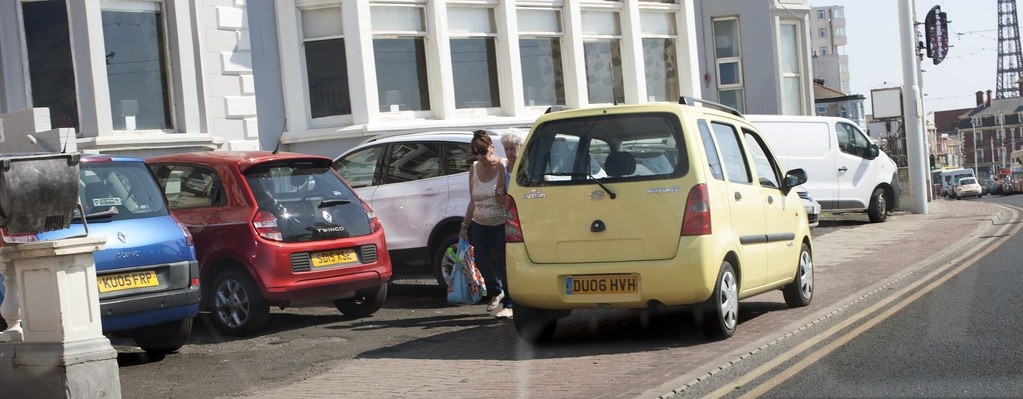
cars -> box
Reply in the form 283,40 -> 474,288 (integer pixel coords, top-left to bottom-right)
955,177 -> 982,199
498,97 -> 818,342
141,150 -> 393,338
932,168 -> 1023,194
0,149 -> 202,356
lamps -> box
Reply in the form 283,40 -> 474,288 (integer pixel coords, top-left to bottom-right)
0,152 -> 89,245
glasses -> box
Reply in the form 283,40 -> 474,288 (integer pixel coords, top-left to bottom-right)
472,146 -> 490,156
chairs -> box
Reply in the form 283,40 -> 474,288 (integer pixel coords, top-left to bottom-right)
604,151 -> 636,178
85,182 -> 131,215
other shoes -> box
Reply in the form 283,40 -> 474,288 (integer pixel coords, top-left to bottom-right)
495,307 -> 514,318
487,289 -> 505,311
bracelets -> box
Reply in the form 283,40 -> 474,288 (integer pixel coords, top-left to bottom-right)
461,223 -> 470,228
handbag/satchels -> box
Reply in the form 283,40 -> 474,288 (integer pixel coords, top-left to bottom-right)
445,233 -> 487,304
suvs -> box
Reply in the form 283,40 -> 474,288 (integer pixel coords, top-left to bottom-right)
289,125 -> 610,295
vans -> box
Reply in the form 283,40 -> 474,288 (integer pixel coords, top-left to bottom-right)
722,113 -> 901,226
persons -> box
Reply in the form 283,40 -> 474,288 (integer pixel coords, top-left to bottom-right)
459,130 -> 515,318
500,133 -> 524,193
